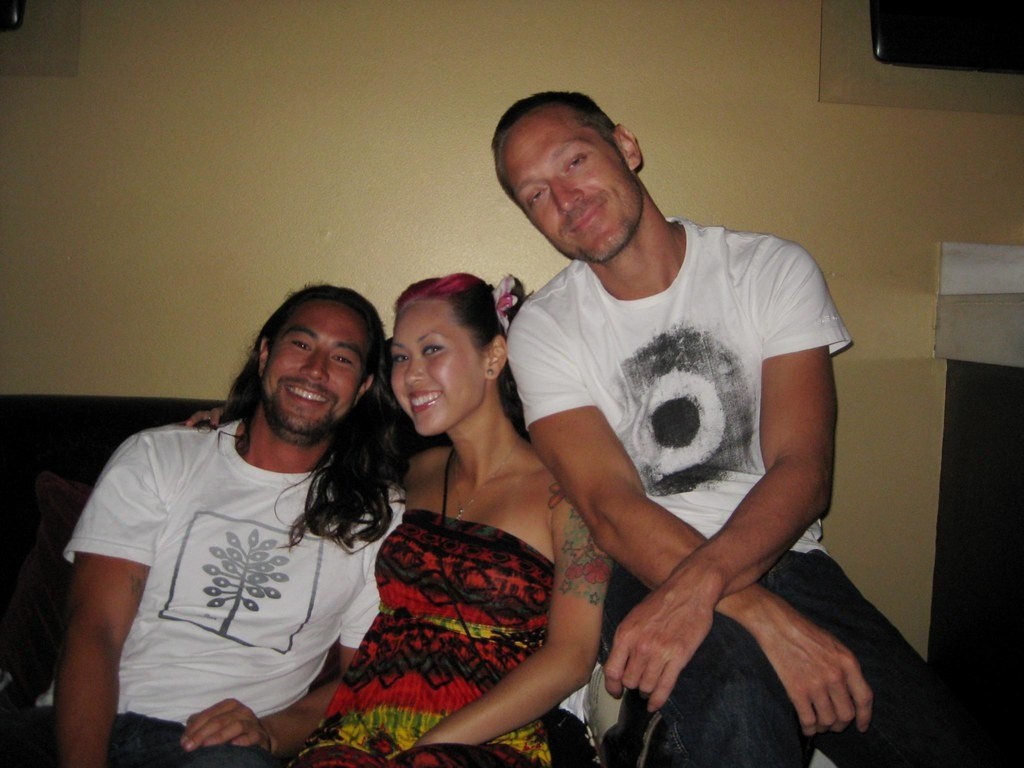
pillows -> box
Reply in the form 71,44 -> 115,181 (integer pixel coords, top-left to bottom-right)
0,470 -> 93,709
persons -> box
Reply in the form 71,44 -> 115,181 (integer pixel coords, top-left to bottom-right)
177,267 -> 608,768
486,92 -> 987,768
49,283 -> 404,768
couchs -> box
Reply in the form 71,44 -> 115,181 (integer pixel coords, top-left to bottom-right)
0,394 -> 669,768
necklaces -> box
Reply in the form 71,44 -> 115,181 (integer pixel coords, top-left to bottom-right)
449,451 -> 520,524
